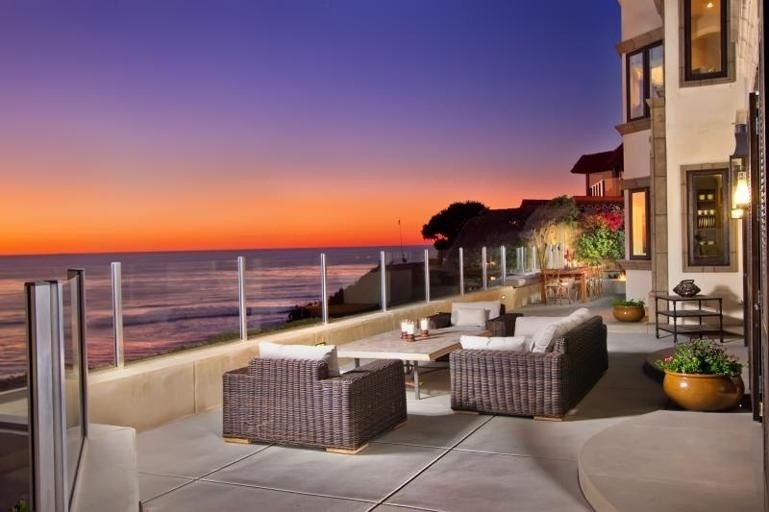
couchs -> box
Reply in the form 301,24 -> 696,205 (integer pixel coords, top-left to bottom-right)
449,316 -> 609,423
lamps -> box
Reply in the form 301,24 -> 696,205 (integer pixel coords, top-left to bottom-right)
729,125 -> 752,219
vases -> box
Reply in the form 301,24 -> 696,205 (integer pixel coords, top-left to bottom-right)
662,369 -> 745,412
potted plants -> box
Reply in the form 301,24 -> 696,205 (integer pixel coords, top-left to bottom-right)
610,297 -> 645,322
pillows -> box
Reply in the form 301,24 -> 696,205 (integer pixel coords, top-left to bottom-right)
459,335 -> 530,352
453,308 -> 486,327
257,342 -> 341,377
532,317 -> 577,353
567,307 -> 591,324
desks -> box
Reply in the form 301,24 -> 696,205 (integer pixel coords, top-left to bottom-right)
655,295 -> 724,345
542,263 -> 605,306
336,326 -> 493,400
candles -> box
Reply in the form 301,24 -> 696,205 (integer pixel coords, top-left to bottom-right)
401,319 -> 408,332
421,317 -> 430,330
406,320 -> 414,334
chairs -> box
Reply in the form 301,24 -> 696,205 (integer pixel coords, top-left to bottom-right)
544,269 -> 570,305
222,335 -> 407,454
417,301 -> 524,337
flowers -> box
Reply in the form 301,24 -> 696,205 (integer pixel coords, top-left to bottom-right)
654,334 -> 750,377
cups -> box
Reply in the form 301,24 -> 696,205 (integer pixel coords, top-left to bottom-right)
417,317 -> 430,338
699,194 -> 713,200
398,319 -> 408,340
698,208 -> 714,215
406,320 -> 417,342
